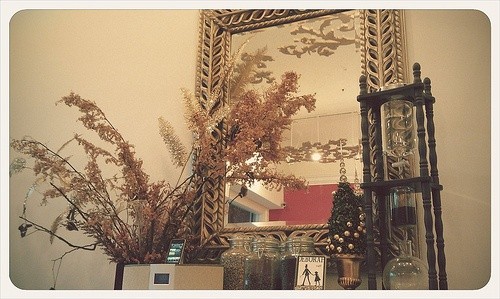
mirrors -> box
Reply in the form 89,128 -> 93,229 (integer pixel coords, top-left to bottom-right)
182,9 -> 423,275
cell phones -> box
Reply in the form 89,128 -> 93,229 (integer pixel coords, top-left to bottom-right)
165,238 -> 187,265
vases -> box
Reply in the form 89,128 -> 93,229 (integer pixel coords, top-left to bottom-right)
113,262 -> 139,290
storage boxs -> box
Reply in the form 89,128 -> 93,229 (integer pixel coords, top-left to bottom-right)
218,232 -> 256,290
279,236 -> 316,290
251,236 -> 284,290
